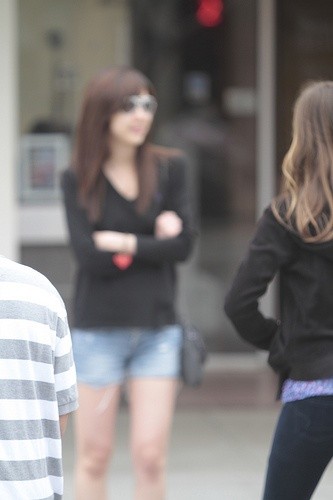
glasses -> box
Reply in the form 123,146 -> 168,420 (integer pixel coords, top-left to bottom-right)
113,95 -> 157,119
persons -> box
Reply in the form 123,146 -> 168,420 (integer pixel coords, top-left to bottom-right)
58,64 -> 200,499
1,253 -> 82,500
220,78 -> 332,500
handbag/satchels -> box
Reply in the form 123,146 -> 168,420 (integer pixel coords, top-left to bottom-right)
180,329 -> 207,388
